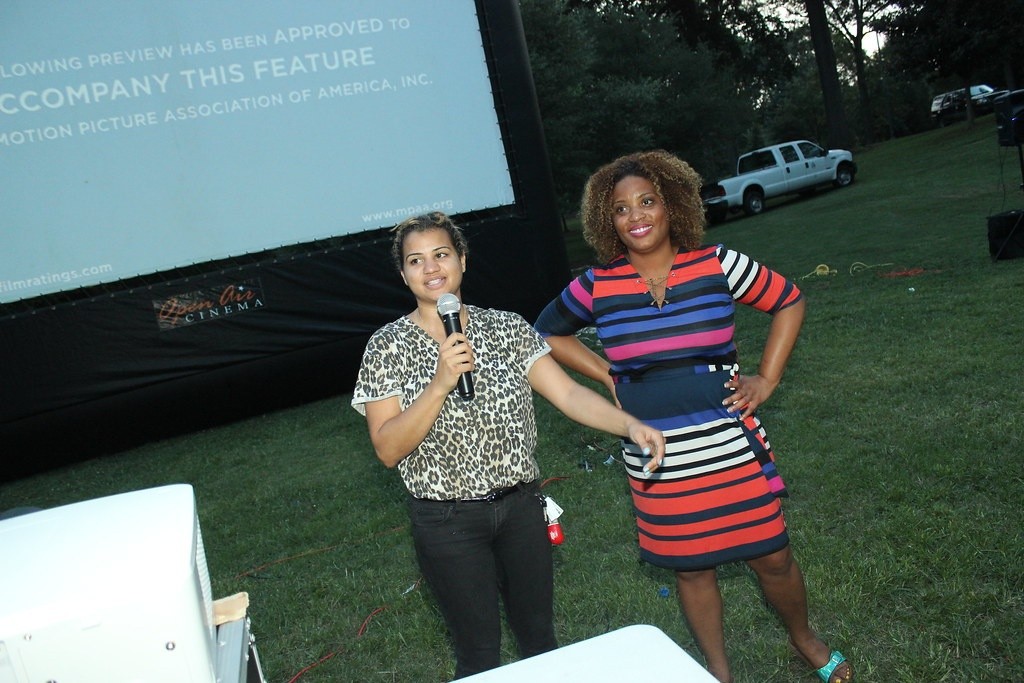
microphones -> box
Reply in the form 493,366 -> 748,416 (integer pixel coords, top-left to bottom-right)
437,294 -> 475,401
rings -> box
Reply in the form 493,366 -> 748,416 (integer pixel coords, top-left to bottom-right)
657,459 -> 662,467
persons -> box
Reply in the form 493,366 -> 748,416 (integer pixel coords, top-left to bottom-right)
351,211 -> 665,680
534,151 -> 854,683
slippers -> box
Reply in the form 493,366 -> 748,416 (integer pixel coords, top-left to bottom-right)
786,639 -> 853,683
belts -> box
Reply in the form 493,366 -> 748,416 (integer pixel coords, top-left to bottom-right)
418,483 -> 519,503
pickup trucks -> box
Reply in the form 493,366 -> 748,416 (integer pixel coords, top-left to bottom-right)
699,139 -> 858,226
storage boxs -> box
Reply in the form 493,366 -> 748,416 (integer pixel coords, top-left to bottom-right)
1,485 -> 268,683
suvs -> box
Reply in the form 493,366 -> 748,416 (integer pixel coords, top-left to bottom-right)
930,84 -> 1010,128
